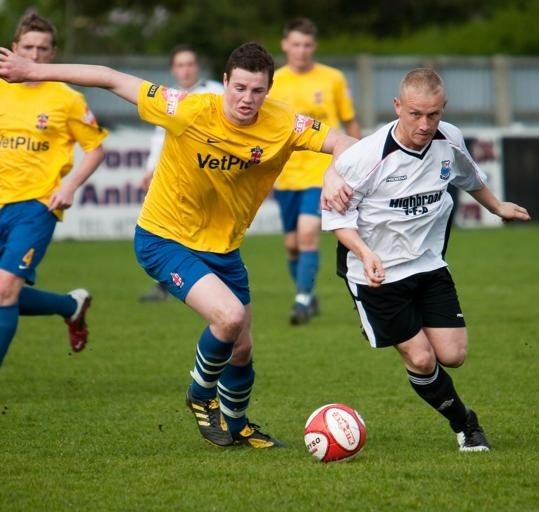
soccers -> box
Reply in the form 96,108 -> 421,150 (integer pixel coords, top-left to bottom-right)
304,404 -> 368,464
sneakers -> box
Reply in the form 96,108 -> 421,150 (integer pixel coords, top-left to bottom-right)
63,286 -> 92,354
229,421 -> 283,448
287,295 -> 319,326
454,409 -> 492,453
185,384 -> 235,448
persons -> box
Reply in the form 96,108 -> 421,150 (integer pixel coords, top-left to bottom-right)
0,40 -> 355,450
136,46 -> 232,304
1,13 -> 107,369
321,68 -> 531,454
265,18 -> 361,326
